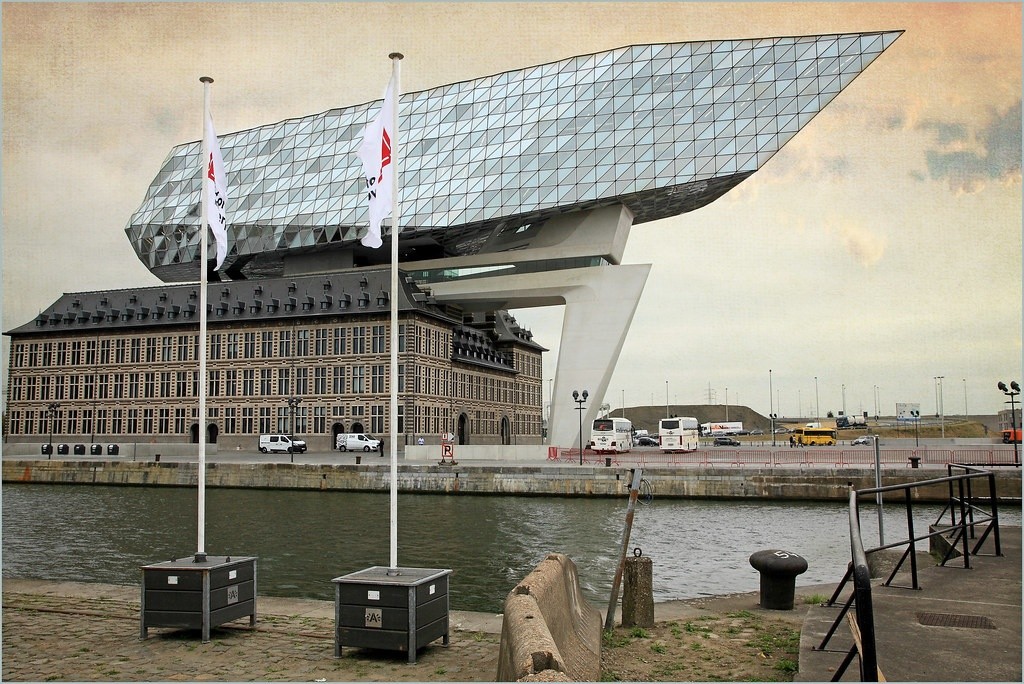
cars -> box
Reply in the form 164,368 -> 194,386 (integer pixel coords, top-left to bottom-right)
709,430 -> 764,436
773,428 -> 795,434
633,434 -> 659,446
854,436 -> 870,444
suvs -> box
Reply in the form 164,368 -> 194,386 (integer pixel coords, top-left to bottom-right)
713,437 -> 740,447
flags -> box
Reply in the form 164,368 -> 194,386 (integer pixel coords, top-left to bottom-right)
206,114 -> 228,271
355,74 -> 394,250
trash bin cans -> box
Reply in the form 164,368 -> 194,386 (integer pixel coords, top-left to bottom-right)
41,444 -> 53,454
58,444 -> 69,454
107,444 -> 119,455
74,444 -> 85,455
91,444 -> 102,455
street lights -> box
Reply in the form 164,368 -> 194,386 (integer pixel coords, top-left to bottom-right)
549,379 -> 553,415
815,377 -> 819,427
666,381 -> 669,418
572,390 -> 588,465
777,390 -> 779,418
999,381 -> 1020,468
934,377 -> 939,417
48,403 -> 61,459
726,388 -> 728,421
911,411 -> 919,447
769,369 -> 772,412
842,384 -> 846,416
622,390 -> 625,418
963,378 -> 968,421
937,377 -> 945,438
877,387 -> 880,419
769,414 -> 777,446
288,396 -> 301,462
798,390 -> 801,423
874,385 -> 877,423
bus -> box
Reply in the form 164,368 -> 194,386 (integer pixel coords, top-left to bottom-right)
591,417 -> 636,454
795,428 -> 838,446
659,417 -> 702,454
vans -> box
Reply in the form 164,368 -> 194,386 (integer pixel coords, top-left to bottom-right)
258,434 -> 307,454
336,433 -> 380,452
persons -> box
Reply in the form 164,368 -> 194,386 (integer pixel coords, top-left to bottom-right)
377,438 -> 384,457
418,436 -> 424,445
790,434 -> 796,448
797,435 -> 804,448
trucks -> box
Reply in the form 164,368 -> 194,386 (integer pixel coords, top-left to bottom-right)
836,416 -> 867,426
701,421 -> 743,436
806,422 -> 837,428
1001,430 -> 1022,444
